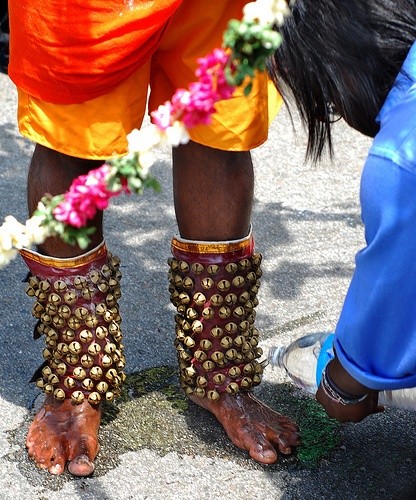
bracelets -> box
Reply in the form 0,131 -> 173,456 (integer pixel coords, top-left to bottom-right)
320,361 -> 380,406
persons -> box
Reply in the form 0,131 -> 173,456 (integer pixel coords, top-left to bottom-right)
265,0 -> 415,424
0,0 -> 301,475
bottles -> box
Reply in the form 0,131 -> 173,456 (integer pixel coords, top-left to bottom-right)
268,330 -> 416,414
